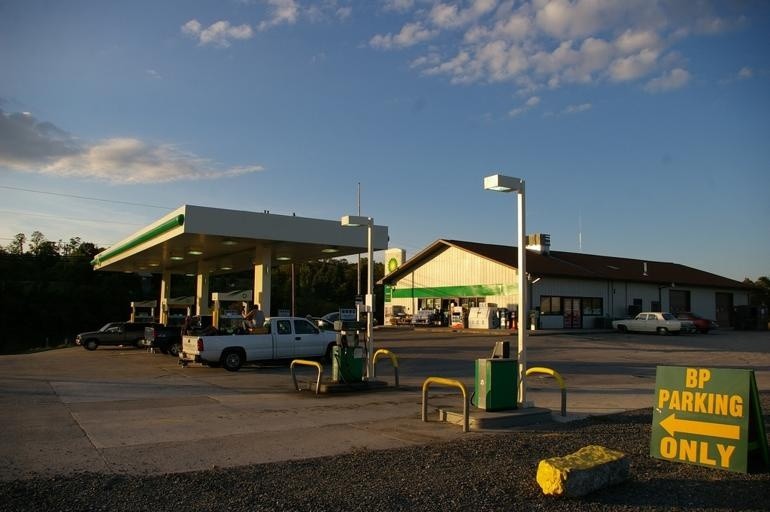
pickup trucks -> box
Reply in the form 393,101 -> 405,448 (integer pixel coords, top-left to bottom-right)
612,310 -> 681,336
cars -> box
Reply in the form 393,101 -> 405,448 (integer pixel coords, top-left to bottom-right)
668,309 -> 721,335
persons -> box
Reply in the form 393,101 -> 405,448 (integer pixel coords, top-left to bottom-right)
242,304 -> 265,332
179,316 -> 194,368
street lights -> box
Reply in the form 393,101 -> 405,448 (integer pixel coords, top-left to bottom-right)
483,172 -> 537,409
341,212 -> 380,383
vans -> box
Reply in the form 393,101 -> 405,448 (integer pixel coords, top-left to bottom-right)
728,302 -> 770,330
